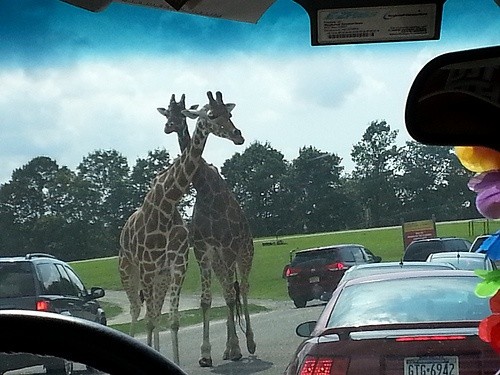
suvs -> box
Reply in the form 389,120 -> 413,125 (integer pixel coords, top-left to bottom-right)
401,236 -> 472,262
0,253 -> 107,327
282,244 -> 382,308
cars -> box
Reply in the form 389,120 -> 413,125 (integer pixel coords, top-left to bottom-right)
469,235 -> 492,253
337,261 -> 456,287
282,270 -> 500,375
426,252 -> 497,271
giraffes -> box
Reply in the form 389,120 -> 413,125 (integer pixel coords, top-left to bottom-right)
156,94 -> 258,367
116,90 -> 245,366
